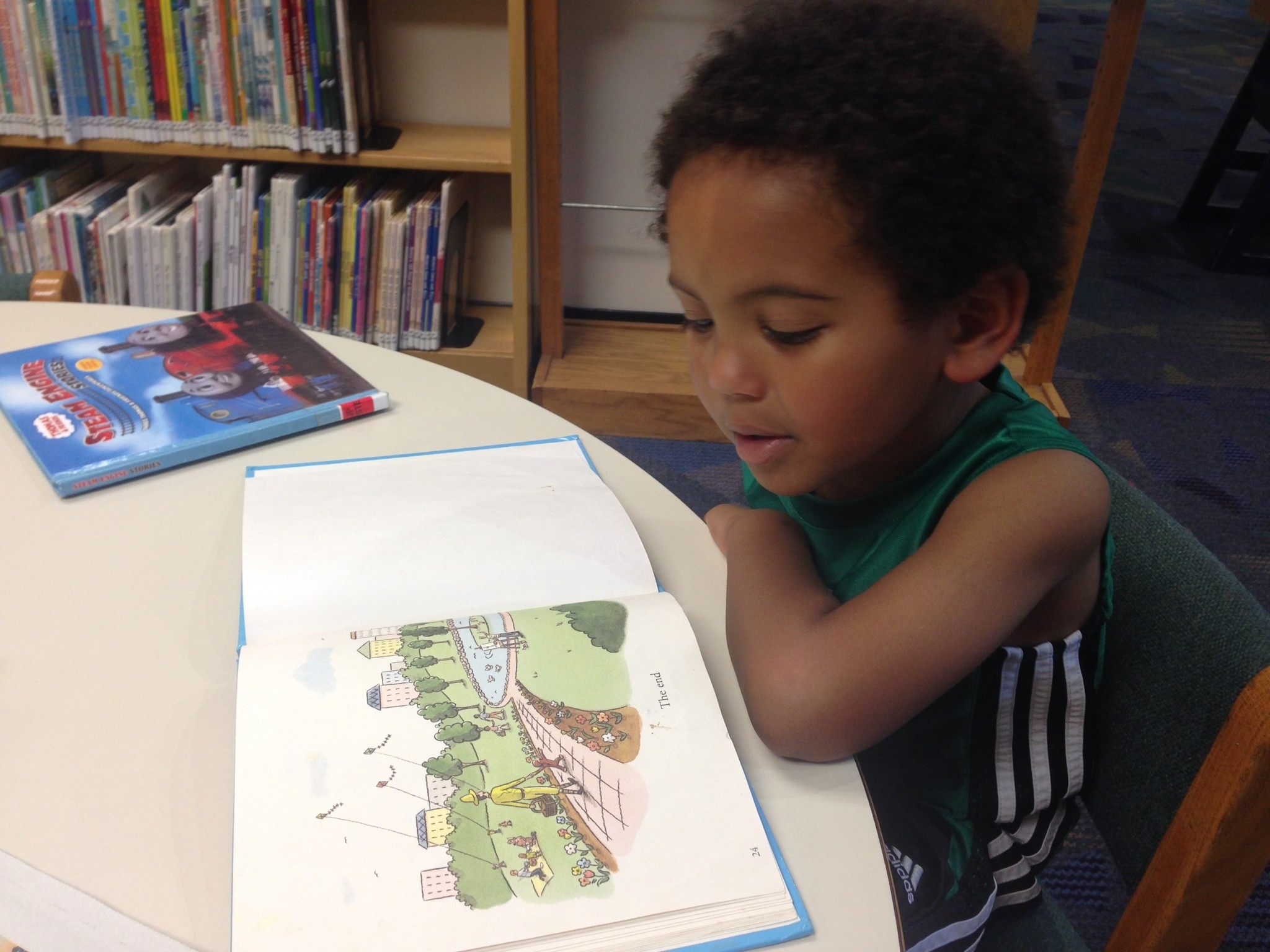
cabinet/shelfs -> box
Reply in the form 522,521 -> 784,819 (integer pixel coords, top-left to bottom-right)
1,0 -> 532,400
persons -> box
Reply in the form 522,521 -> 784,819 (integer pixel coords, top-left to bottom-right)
647,0 -> 1270,952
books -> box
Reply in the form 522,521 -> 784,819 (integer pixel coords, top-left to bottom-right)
0,151 -> 472,351
0,0 -> 384,156
0,297 -> 391,501
233,434 -> 817,952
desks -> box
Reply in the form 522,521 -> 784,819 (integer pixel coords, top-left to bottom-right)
1,298 -> 905,951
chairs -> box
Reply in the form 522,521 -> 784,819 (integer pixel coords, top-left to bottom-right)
974,460 -> 1270,952
